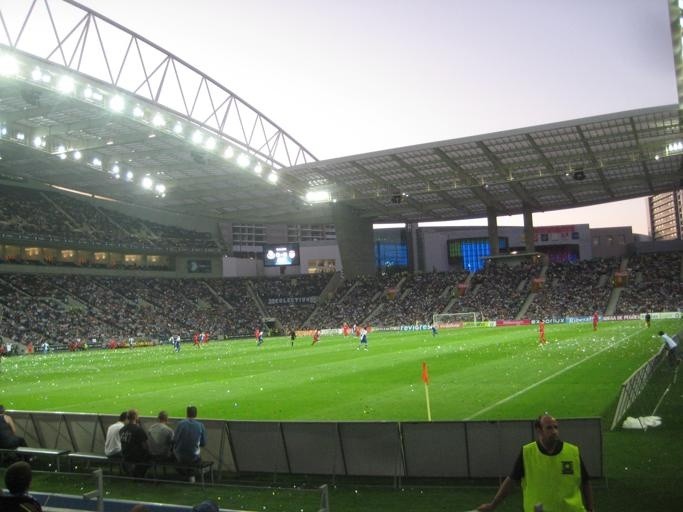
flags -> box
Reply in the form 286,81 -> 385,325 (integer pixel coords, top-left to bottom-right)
420,362 -> 430,386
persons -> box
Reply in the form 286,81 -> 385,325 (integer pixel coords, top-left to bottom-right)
1,459 -> 44,511
1,272 -> 683,359
1,256 -> 172,272
657,330 -> 681,366
192,498 -> 220,511
170,404 -> 209,484
1,404 -> 37,462
118,408 -> 151,476
476,413 -> 594,511
144,408 -> 183,480
0,185 -> 222,250
103,410 -> 132,472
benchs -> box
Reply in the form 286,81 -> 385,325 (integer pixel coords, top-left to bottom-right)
0,446 -> 215,492
